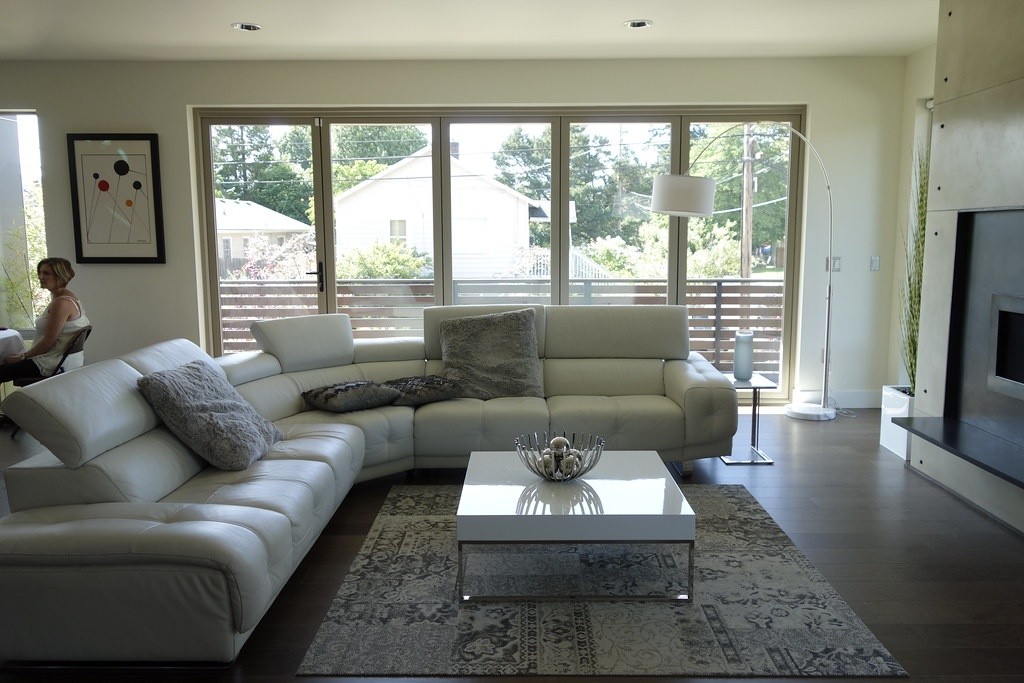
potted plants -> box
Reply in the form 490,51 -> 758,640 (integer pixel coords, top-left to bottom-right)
879,142 -> 931,460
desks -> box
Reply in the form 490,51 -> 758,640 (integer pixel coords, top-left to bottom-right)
0,329 -> 19,357
453,451 -> 695,604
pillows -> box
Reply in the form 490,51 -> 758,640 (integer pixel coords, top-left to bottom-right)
301,380 -> 401,413
137,359 -> 282,472
440,307 -> 546,399
384,375 -> 464,406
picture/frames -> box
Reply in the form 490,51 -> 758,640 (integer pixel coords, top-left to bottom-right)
66,132 -> 166,265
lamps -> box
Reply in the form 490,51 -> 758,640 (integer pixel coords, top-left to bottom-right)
650,121 -> 836,421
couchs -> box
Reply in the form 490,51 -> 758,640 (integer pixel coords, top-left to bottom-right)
0,304 -> 739,662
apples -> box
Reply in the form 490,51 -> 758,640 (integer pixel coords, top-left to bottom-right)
537,436 -> 583,476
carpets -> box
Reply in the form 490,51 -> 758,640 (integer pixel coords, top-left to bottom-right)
296,485 -> 911,675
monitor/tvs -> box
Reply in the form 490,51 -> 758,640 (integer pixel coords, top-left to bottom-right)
987,294 -> 1024,401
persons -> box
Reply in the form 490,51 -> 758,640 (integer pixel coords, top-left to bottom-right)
0,257 -> 90,383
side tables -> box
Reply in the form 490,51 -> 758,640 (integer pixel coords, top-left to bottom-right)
720,373 -> 778,465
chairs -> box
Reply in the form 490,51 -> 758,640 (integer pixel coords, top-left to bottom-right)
13,325 -> 92,387
